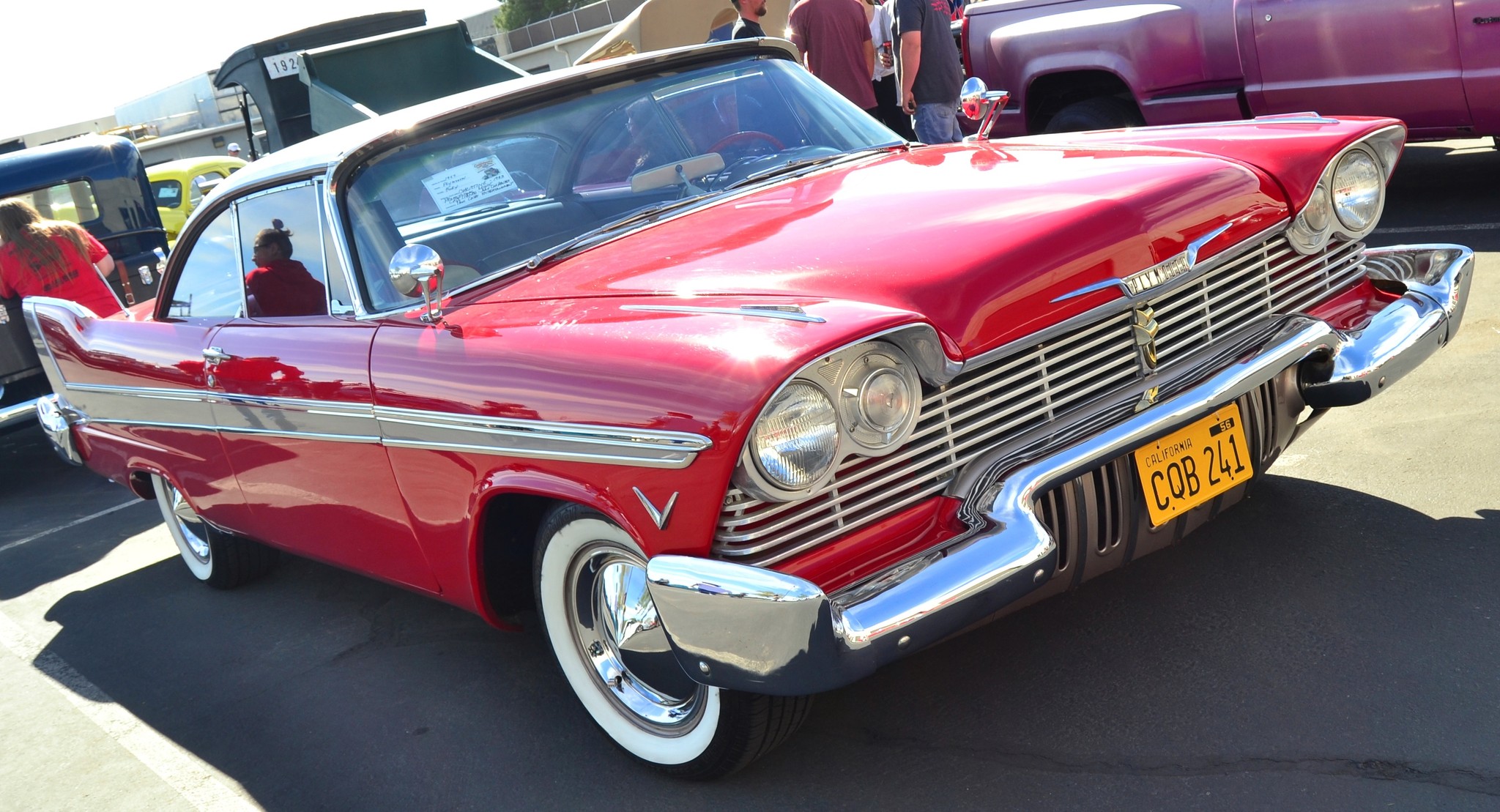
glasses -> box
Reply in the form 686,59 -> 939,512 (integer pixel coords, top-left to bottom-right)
253,244 -> 268,252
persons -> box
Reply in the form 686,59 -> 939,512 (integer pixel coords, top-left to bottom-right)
732,0 -> 803,154
786,0 -> 881,151
892,0 -> 968,144
235,219 -> 328,316
876,0 -> 917,144
625,96 -> 700,188
0,197 -> 124,326
946,0 -> 970,63
226,142 -> 242,158
246,149 -> 261,163
854,0 -> 916,140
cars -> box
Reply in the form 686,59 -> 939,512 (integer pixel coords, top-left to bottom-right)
21,36 -> 1475,781
144,155 -> 251,254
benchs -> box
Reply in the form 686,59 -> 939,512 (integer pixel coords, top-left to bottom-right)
402,195 -> 599,276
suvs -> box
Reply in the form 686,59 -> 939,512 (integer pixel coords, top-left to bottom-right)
0,133 -> 170,431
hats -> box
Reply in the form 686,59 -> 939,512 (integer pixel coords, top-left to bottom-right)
227,143 -> 240,152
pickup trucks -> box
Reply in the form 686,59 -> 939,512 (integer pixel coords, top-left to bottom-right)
965,0 -> 1500,144
